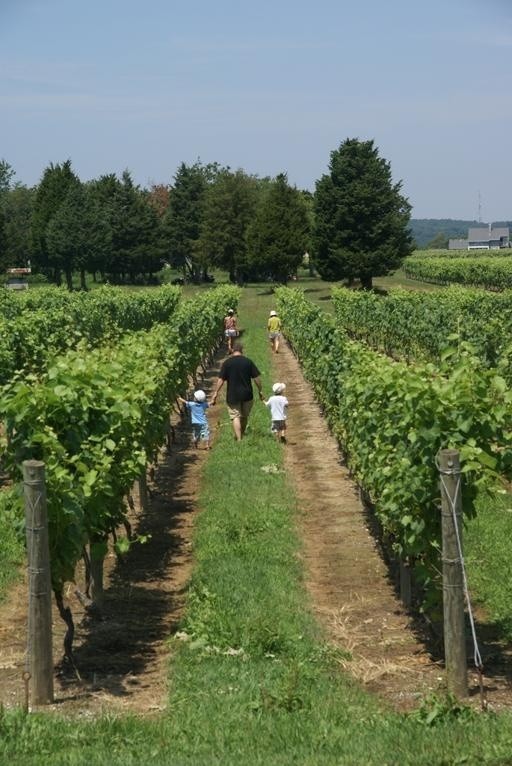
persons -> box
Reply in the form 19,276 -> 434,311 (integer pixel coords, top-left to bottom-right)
262,382 -> 289,444
268,310 -> 282,354
211,344 -> 265,442
178,389 -> 215,450
223,308 -> 240,354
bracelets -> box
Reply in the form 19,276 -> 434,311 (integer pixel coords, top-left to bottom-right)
259,392 -> 263,395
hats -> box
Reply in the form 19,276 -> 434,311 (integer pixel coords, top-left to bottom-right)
272,383 -> 286,394
194,390 -> 206,403
269,310 -> 277,317
